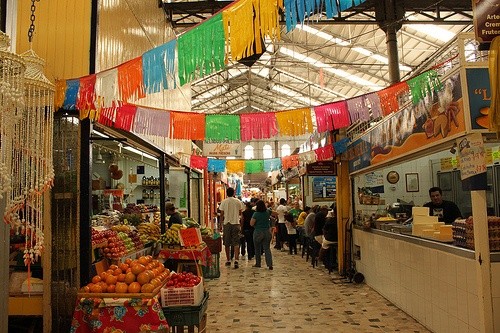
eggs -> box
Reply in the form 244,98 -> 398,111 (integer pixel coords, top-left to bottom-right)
465,215 -> 500,251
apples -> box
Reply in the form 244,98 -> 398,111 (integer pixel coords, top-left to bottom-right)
92,227 -> 128,257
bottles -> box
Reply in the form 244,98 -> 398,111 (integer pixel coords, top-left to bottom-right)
142,176 -> 160,185
142,187 -> 154,199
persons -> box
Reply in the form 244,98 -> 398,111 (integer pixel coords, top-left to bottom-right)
249,201 -> 278,270
166,202 -> 183,229
237,196 -> 338,260
219,188 -> 242,269
423,188 -> 462,224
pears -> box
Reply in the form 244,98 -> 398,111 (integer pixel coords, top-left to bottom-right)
117,231 -> 135,252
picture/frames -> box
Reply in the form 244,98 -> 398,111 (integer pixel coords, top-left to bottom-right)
387,170 -> 400,184
405,172 -> 420,193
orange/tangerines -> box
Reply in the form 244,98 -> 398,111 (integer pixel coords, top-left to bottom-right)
81,254 -> 170,293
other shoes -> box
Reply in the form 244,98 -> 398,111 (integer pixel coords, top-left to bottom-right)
225,244 -> 322,270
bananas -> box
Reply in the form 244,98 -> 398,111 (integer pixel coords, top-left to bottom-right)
139,222 -> 188,244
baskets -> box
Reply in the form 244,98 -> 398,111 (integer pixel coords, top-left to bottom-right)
161,275 -> 204,307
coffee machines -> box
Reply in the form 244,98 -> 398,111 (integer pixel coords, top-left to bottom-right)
391,202 -> 417,223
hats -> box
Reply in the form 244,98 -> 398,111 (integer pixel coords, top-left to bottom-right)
321,205 -> 332,211
165,202 -> 174,210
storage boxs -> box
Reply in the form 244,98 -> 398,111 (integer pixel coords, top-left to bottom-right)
158,274 -> 210,328
